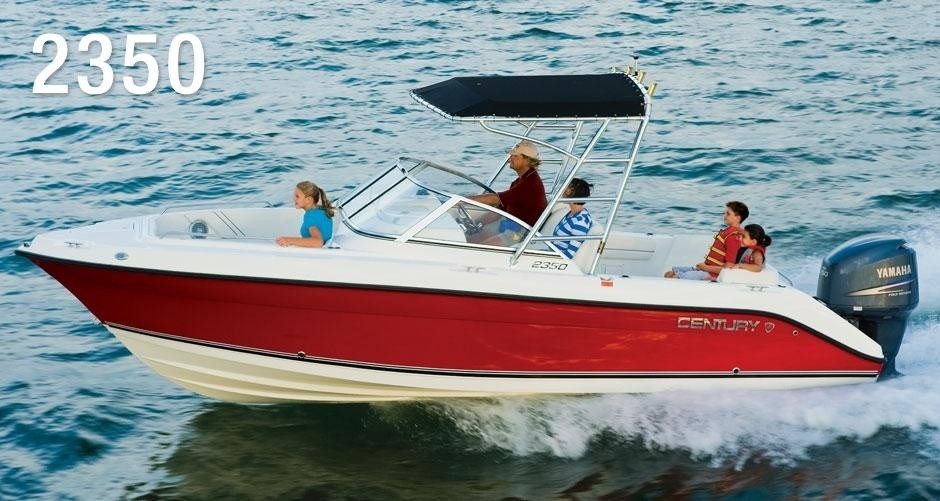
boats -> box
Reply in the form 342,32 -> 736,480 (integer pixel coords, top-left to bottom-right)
8,61 -> 920,409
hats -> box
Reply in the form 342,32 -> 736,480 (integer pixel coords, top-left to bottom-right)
506,142 -> 540,160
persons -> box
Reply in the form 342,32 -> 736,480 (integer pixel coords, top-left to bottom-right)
705,223 -> 772,282
276,180 -> 336,250
460,140 -> 551,248
509,177 -> 595,262
663,201 -> 749,281
